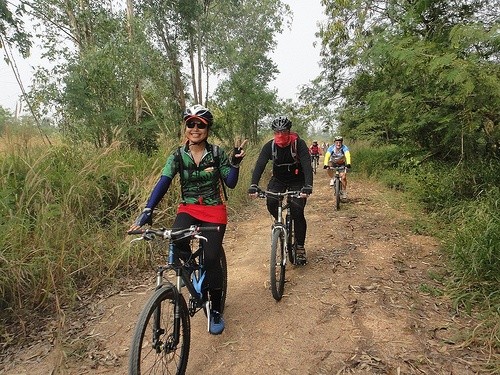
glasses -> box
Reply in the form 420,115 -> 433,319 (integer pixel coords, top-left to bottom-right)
187,121 -> 206,129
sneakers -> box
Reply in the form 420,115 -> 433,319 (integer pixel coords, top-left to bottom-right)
295,244 -> 306,265
209,309 -> 225,335
181,263 -> 196,286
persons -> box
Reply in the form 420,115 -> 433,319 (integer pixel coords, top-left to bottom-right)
247,116 -> 313,266
322,142 -> 326,150
309,140 -> 322,172
323,136 -> 351,199
128,105 -> 249,334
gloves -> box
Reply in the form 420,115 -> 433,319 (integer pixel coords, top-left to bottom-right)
324,166 -> 329,169
346,165 -> 351,168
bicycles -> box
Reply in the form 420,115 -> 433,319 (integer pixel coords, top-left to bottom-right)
311,154 -> 319,175
247,186 -> 312,301
127,223 -> 228,375
324,166 -> 348,211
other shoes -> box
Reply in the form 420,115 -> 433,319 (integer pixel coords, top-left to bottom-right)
342,192 -> 346,198
330,177 -> 336,185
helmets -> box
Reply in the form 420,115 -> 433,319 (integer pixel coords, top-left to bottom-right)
272,116 -> 292,131
184,104 -> 213,126
312,140 -> 317,143
334,137 -> 343,142
